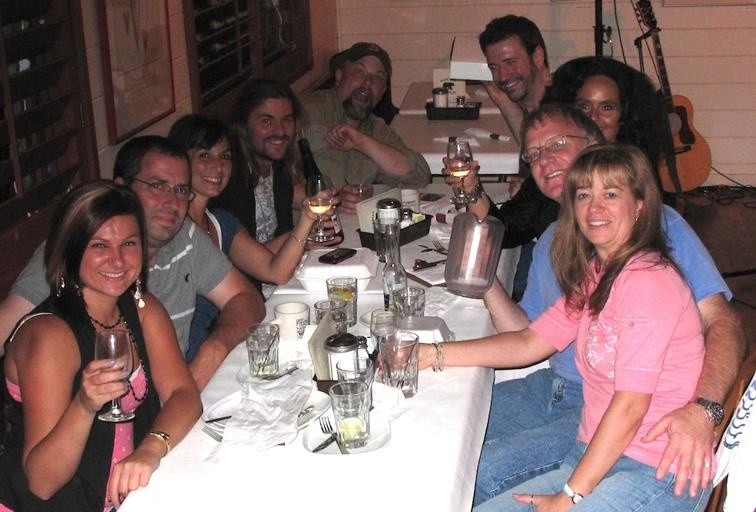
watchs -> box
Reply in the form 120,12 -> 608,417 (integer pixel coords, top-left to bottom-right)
691,396 -> 724,428
561,483 -> 583,505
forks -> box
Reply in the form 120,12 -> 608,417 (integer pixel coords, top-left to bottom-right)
432,240 -> 448,252
318,416 -> 348,454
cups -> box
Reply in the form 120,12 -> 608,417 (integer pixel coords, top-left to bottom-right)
350,175 -> 372,216
314,300 -> 348,333
336,357 -> 375,412
331,382 -> 371,450
380,331 -> 419,397
270,300 -> 310,345
249,321 -> 280,376
324,276 -> 358,327
401,189 -> 420,214
390,285 -> 428,319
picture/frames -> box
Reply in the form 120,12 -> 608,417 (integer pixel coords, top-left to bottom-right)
95,0 -> 176,145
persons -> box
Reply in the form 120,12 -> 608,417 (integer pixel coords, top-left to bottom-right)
377,145 -> 713,512
167,113 -> 337,366
478,13 -> 549,147
442,54 -> 665,250
0,135 -> 266,393
202,78 -> 373,257
272,42 -> 431,188
0,181 -> 203,512
474,100 -> 747,506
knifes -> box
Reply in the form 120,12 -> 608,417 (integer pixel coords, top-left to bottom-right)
312,433 -> 340,453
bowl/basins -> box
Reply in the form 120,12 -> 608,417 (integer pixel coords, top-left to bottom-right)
295,247 -> 379,293
378,315 -> 455,344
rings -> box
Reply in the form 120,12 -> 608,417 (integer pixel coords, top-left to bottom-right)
530,495 -> 533,505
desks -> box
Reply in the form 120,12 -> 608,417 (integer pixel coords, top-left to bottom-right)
114,292 -> 494,512
274,184 -> 521,307
399,82 -> 508,114
389,114 -> 522,183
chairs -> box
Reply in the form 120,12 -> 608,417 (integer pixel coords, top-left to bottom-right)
700,350 -> 756,512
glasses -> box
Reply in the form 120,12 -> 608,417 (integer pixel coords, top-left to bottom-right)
521,135 -> 587,163
131,176 -> 189,195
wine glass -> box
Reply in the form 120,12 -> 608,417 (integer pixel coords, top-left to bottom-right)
94,329 -> 136,423
447,141 -> 478,205
306,176 -> 334,242
370,307 -> 398,383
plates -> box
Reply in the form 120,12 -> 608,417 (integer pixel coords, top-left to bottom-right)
202,389 -> 331,433
360,310 -> 372,328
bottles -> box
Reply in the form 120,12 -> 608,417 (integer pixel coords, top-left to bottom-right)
432,88 -> 448,108
382,225 -> 408,313
456,96 -> 465,108
377,198 -> 401,223
401,209 -> 415,229
297,138 -> 344,246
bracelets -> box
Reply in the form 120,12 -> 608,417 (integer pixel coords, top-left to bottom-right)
290,234 -> 308,244
143,431 -> 171,457
432,342 -> 444,372
464,181 -> 485,205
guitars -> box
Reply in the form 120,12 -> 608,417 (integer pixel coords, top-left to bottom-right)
636,0 -> 712,194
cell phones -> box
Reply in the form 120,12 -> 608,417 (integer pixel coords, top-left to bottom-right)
318,247 -> 357,264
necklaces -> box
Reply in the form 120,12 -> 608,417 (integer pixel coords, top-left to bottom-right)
186,213 -> 210,235
89,314 -> 149,401
